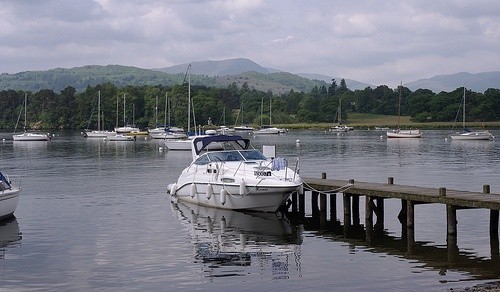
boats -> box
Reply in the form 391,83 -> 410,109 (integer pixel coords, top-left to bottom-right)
166,133 -> 303,214
0,168 -> 21,221
163,98 -> 233,150
107,134 -> 136,141
375,126 -> 389,131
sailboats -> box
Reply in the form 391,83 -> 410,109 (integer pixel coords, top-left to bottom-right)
387,81 -> 423,138
328,99 -> 354,132
11,93 -> 51,141
82,90 -> 117,138
114,66 -> 289,139
448,87 -> 497,141
